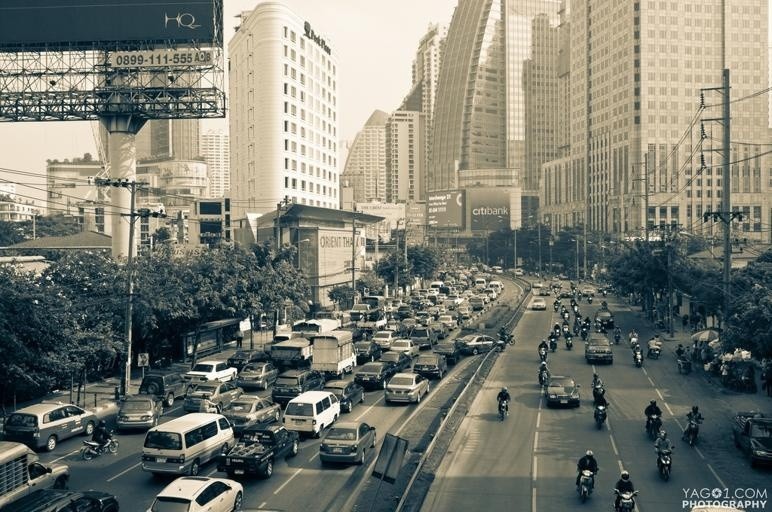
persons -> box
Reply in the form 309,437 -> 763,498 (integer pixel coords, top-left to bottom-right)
493,257 -> 771,512
90,418 -> 113,454
235,328 -> 245,349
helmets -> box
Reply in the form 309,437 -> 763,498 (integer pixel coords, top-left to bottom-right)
650,400 -> 656,406
692,406 -> 698,413
620,471 -> 630,483
659,430 -> 667,440
585,450 -> 594,460
502,387 -> 507,392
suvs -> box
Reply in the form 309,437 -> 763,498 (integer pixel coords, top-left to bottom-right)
321,379 -> 367,414
585,333 -> 613,363
138,370 -> 194,408
409,328 -> 439,349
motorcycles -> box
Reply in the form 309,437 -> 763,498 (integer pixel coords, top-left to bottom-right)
573,463 -> 599,502
588,298 -> 592,303
655,340 -> 662,347
592,403 -> 611,426
536,369 -> 550,384
548,337 -> 557,352
498,399 -> 508,421
578,294 -> 582,301
596,328 -> 607,336
563,335 -> 574,350
81,428 -> 120,460
630,332 -> 638,347
538,347 -> 547,360
601,303 -> 608,310
590,381 -> 606,402
632,349 -> 645,366
647,342 -> 659,359
655,446 -> 675,480
676,346 -> 691,375
646,409 -> 661,441
500,334 -> 515,346
685,414 -> 704,447
552,298 -> 591,340
614,329 -> 620,345
613,489 -> 639,511
603,290 -> 607,296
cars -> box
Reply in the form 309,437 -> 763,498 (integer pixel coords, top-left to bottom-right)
185,358 -> 236,384
320,421 -> 376,466
598,285 -> 616,293
183,377 -> 244,414
532,298 -> 546,310
221,394 -> 282,432
354,340 -> 382,364
229,347 -> 270,370
431,343 -> 458,364
391,340 -> 420,358
370,330 -> 401,349
238,363 -> 278,392
0,488 -> 120,511
415,352 -> 448,379
313,263 -> 504,337
508,268 -> 595,297
143,475 -> 244,512
594,309 -> 616,329
545,375 -> 581,406
379,352 -> 410,370
454,335 -> 505,356
385,372 -> 429,403
115,395 -> 163,429
353,362 -> 398,389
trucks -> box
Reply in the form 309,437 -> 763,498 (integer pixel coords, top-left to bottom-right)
0,439 -> 68,508
309,329 -> 357,379
270,330 -> 322,371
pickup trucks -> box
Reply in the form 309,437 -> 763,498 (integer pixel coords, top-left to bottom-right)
731,410 -> 772,468
216,424 -> 302,479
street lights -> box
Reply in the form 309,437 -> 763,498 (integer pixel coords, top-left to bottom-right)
570,238 -> 624,286
470,215 -> 503,265
653,222 -> 684,336
529,214 -> 551,278
498,214 -> 533,270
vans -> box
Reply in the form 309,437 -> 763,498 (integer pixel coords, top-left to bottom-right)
272,367 -> 325,404
143,412 -> 236,477
272,324 -> 292,343
5,400 -> 99,451
281,391 -> 342,438
